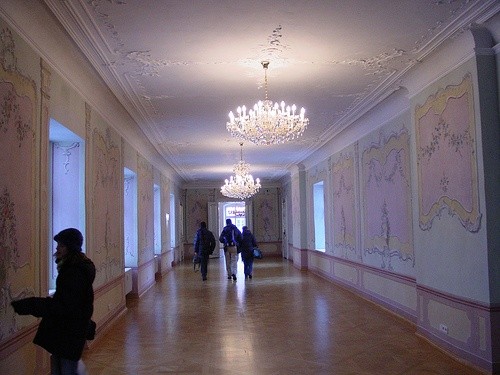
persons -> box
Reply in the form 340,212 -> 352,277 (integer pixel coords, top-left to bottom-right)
240,226 -> 258,280
9,228 -> 96,375
219,219 -> 242,281
194,221 -> 216,281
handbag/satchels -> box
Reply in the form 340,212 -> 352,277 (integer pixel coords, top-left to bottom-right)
192,256 -> 201,272
249,247 -> 264,259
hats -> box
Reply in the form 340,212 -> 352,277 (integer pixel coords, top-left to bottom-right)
54,228 -> 83,251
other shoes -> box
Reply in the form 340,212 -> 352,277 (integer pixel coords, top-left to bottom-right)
228,274 -> 237,281
245,273 -> 252,278
203,276 -> 207,281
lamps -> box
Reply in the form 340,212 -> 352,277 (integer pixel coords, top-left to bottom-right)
219,142 -> 262,200
226,61 -> 309,147
